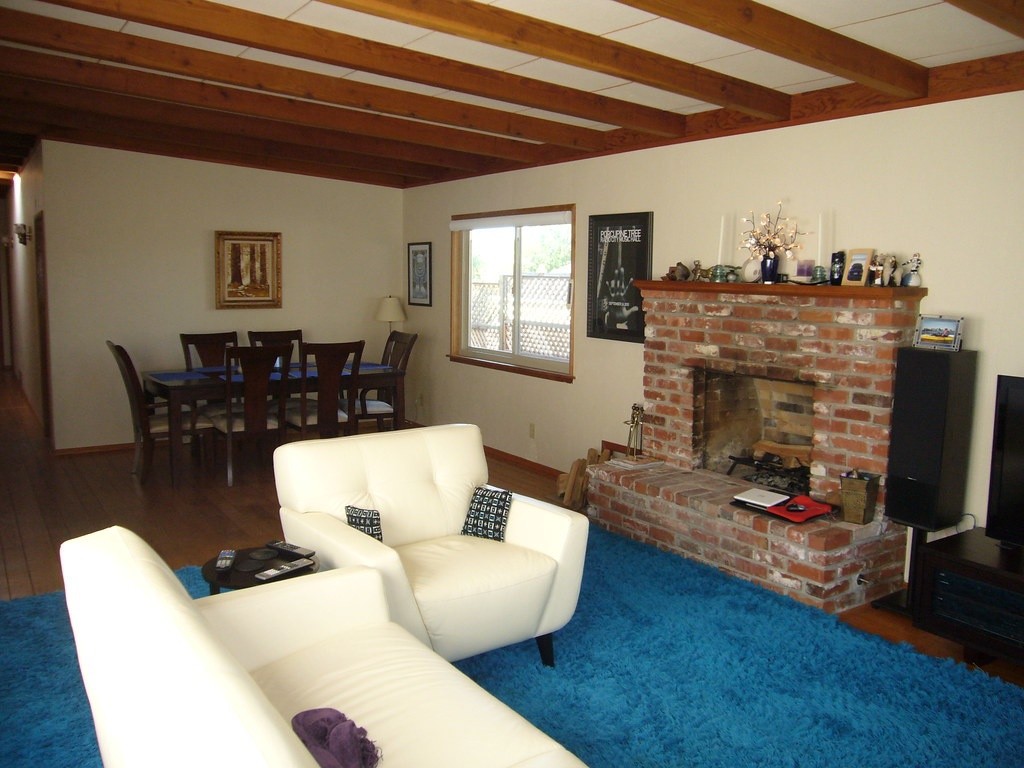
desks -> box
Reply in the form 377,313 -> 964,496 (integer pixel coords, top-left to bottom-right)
201,542 -> 318,595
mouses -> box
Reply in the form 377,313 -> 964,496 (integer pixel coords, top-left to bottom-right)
786,502 -> 806,511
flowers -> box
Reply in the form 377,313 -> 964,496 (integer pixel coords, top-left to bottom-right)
736,202 -> 804,262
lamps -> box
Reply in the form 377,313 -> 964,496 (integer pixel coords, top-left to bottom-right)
378,294 -> 406,340
12,223 -> 29,244
0,234 -> 10,248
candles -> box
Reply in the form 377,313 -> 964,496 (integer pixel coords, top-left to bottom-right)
817,213 -> 822,265
718,216 -> 724,264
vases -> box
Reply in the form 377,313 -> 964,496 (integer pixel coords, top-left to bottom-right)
761,255 -> 779,285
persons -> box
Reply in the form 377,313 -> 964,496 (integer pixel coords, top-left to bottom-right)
868,253 -> 921,286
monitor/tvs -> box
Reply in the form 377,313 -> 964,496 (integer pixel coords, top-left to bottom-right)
986,375 -> 1024,548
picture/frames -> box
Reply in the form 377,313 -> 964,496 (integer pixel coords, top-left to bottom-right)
213,229 -> 284,308
587,211 -> 653,343
408,241 -> 432,306
913,314 -> 964,352
841,248 -> 873,286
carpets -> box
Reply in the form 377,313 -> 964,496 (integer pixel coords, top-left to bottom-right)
0,514 -> 1023,768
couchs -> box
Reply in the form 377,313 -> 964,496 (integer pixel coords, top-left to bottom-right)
58,525 -> 590,767
273,423 -> 590,668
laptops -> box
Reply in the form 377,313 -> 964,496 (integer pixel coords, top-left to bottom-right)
734,487 -> 791,511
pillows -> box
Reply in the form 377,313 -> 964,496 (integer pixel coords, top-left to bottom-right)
460,486 -> 512,542
345,506 -> 383,544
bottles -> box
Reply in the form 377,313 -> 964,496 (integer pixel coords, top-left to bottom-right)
810,266 -> 828,286
726,270 -> 738,282
830,253 -> 845,285
710,264 -> 727,282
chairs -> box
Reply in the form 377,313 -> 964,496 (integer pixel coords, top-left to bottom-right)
104,331 -> 416,487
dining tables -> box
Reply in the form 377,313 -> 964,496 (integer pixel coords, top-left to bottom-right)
142,362 -> 406,490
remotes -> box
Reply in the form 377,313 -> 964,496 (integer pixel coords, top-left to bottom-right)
255,557 -> 313,580
266,540 -> 316,557
215,549 -> 237,572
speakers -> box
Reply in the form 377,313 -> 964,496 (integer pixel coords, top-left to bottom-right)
885,346 -> 978,533
